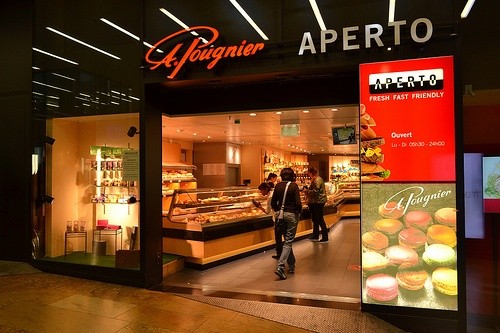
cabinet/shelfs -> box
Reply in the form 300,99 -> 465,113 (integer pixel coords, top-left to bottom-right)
91,160 -> 196,256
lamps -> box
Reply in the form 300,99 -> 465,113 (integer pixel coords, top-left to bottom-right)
41,195 -> 54,204
39,134 -> 55,145
127,126 -> 139,137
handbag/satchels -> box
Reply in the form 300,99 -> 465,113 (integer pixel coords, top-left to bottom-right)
276,207 -> 284,225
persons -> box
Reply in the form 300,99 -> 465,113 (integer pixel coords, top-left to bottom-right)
266,173 -> 280,188
303,167 -> 329,243
258,183 -> 282,259
271,168 -> 302,278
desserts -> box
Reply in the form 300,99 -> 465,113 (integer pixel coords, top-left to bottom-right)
360,202 -> 458,301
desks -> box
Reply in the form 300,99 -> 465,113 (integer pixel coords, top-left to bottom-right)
93,228 -> 122,256
65,231 -> 87,256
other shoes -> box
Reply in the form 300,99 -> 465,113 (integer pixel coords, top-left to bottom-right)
288,265 -> 295,273
308,235 -> 319,240
317,239 -> 328,243
272,255 -> 280,260
274,267 -> 287,279
